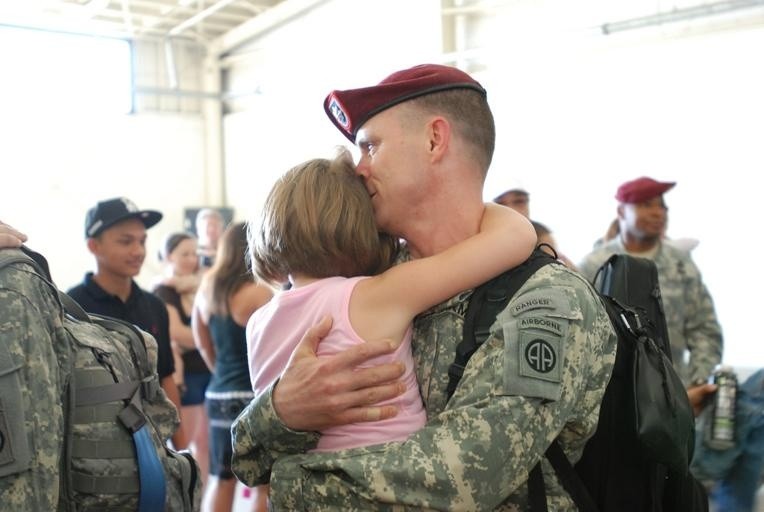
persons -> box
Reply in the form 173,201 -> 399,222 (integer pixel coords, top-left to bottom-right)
150,176 -> 764,512
228,62 -> 616,509
62,199 -> 188,458
1,219 -> 84,511
241,155 -> 541,460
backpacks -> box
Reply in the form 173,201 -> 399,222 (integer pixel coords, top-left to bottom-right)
447,244 -> 708,512
1,243 -> 201,512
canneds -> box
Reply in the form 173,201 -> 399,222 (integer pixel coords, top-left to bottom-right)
702,371 -> 738,451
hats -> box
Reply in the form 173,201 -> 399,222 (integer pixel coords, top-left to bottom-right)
85,198 -> 162,237
615,178 -> 676,204
323,63 -> 487,144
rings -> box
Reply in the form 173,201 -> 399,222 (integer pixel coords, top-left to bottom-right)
366,405 -> 384,426
